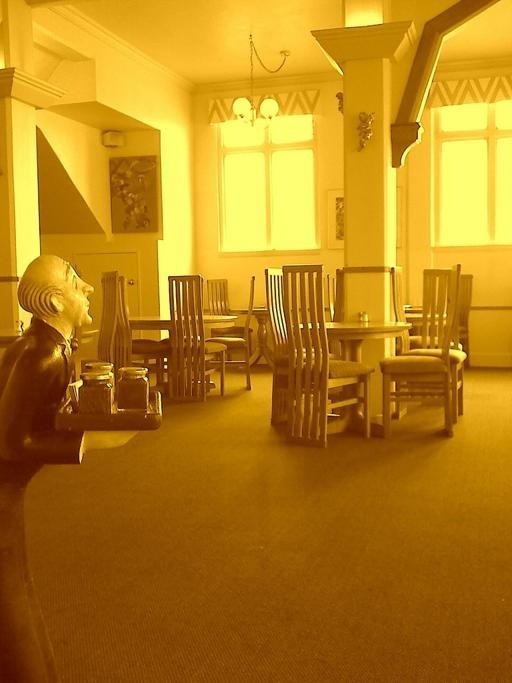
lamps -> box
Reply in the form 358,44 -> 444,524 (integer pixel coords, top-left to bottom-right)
232,31 -> 291,127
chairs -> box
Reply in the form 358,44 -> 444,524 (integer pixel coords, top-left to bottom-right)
204,279 -> 255,370
263,268 -> 339,426
280,263 -> 373,445
390,266 -> 467,424
168,274 -> 227,402
205,273 -> 256,392
403,269 -> 472,376
99,270 -> 163,401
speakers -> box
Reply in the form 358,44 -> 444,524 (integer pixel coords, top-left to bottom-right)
102,131 -> 125,147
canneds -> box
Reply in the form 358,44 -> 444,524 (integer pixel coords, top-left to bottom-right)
77,361 -> 149,415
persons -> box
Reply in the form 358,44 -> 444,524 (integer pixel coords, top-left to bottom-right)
0,253 -> 139,682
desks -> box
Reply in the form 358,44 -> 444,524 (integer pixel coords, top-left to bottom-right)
229,304 -> 306,374
291,320 -> 412,439
378,263 -> 461,439
126,316 -> 238,398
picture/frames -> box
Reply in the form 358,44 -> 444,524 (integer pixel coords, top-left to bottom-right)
325,186 -> 345,249
